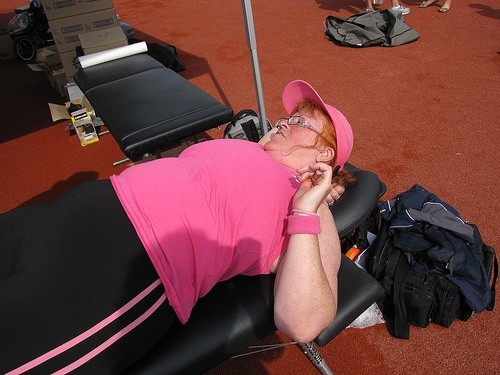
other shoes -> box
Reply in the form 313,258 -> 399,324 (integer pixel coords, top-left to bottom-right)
440,3 -> 452,12
419,0 -> 438,8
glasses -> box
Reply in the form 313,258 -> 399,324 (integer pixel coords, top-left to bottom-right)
276,115 -> 332,147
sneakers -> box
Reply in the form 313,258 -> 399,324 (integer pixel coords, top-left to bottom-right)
391,4 -> 410,16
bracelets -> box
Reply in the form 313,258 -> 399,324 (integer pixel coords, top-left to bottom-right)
287,215 -> 321,235
292,208 -> 322,218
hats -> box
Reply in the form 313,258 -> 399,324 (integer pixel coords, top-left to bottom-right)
281,79 -> 353,172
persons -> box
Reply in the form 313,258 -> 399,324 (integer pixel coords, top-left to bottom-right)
365,0 -> 452,15
0,78 -> 354,375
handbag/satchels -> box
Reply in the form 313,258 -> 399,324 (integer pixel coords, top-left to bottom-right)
355,183 -> 498,341
363,218 -> 497,340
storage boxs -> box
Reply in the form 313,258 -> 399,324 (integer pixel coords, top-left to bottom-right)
77,26 -> 128,55
58,50 -> 82,84
41,0 -> 114,21
47,8 -> 119,53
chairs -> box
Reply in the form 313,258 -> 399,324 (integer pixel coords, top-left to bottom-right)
72,47 -> 234,161
113,162 -> 387,375
